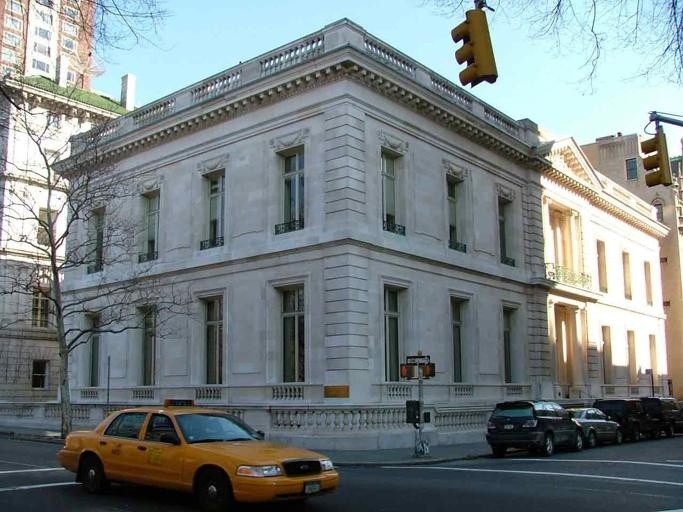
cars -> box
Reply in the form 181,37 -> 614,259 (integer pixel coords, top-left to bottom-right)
564,407 -> 625,448
56,398 -> 340,510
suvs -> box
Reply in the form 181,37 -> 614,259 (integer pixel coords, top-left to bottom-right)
592,396 -> 683,442
485,398 -> 588,457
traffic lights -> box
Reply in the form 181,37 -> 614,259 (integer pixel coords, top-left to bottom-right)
422,363 -> 436,377
400,362 -> 416,377
406,400 -> 419,423
640,132 -> 673,188
450,8 -> 498,88
